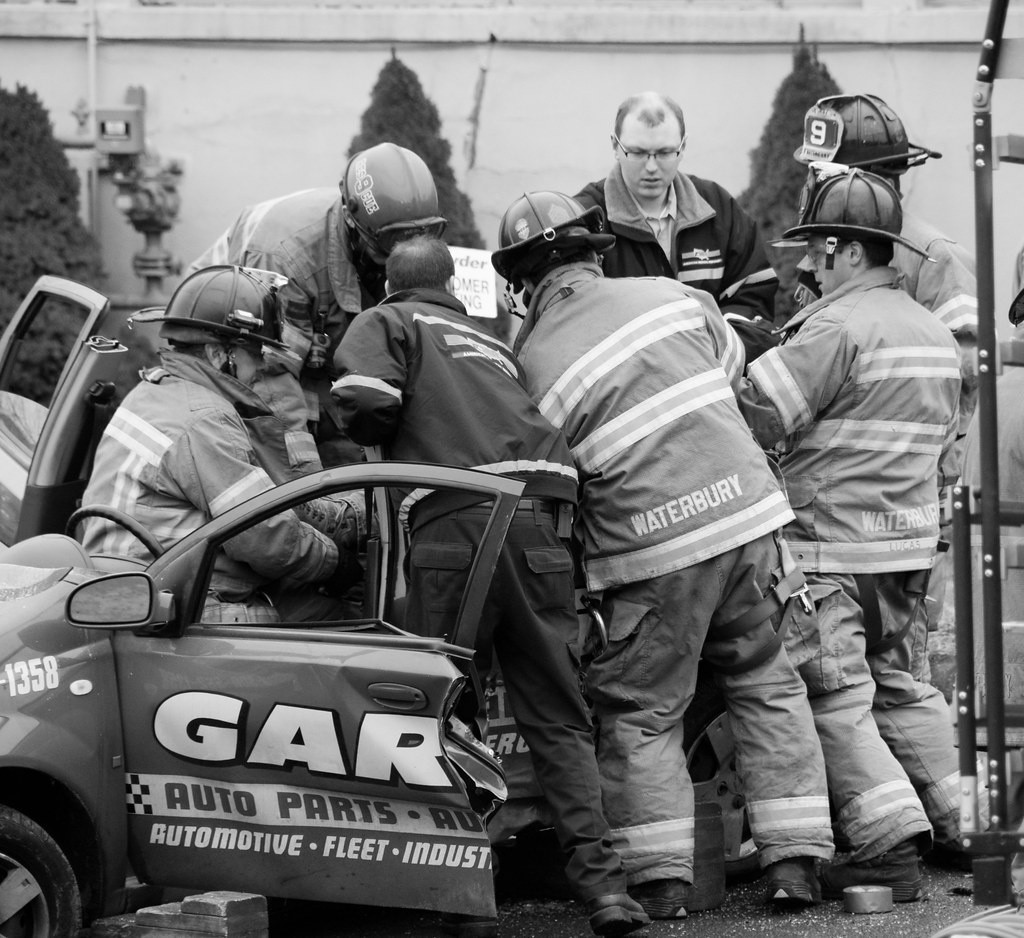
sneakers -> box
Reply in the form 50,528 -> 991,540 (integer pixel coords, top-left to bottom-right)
766,852 -> 824,903
632,880 -> 692,919
818,844 -> 925,901
589,890 -> 650,934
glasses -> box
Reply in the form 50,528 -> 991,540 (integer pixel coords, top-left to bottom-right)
614,133 -> 686,161
804,242 -> 852,263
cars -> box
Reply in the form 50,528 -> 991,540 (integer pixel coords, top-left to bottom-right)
1,275 -> 769,937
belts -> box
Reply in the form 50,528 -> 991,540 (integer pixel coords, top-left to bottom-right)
477,500 -> 556,511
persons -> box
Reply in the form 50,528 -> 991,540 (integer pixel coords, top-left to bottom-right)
326,232 -> 655,938
199,264 -> 382,627
180,139 -> 449,474
733,166 -> 975,904
778,92 -> 980,686
489,189 -> 837,920
497,91 -> 779,381
78,264 -> 364,627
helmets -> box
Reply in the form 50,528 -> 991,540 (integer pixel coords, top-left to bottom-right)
792,93 -> 908,174
1008,243 -> 1024,328
339,142 -> 439,256
492,188 -> 606,286
129,264 -> 292,351
781,169 -> 930,260
